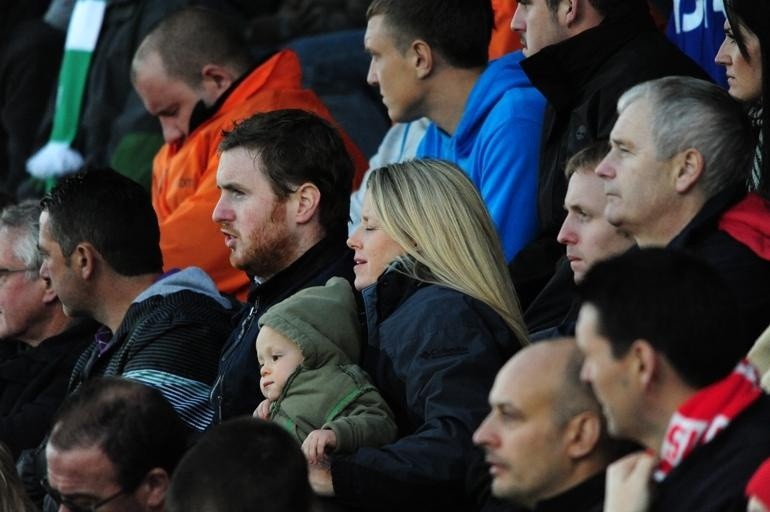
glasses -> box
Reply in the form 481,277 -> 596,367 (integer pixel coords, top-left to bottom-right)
0,268 -> 36,278
40,475 -> 128,512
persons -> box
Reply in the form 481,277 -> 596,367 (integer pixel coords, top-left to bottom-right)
210,109 -> 355,425
363,1 -> 546,268
512,1 -> 716,157
44,379 -> 190,511
132,8 -> 366,303
38,163 -> 244,512
173,417 -> 316,512
0,200 -> 112,509
557,144 -> 635,279
473,337 -> 607,511
721,3 -> 768,196
597,76 -> 767,287
312,158 -> 533,509
253,274 -> 398,456
1,0 -> 179,189
575,245 -> 768,512
